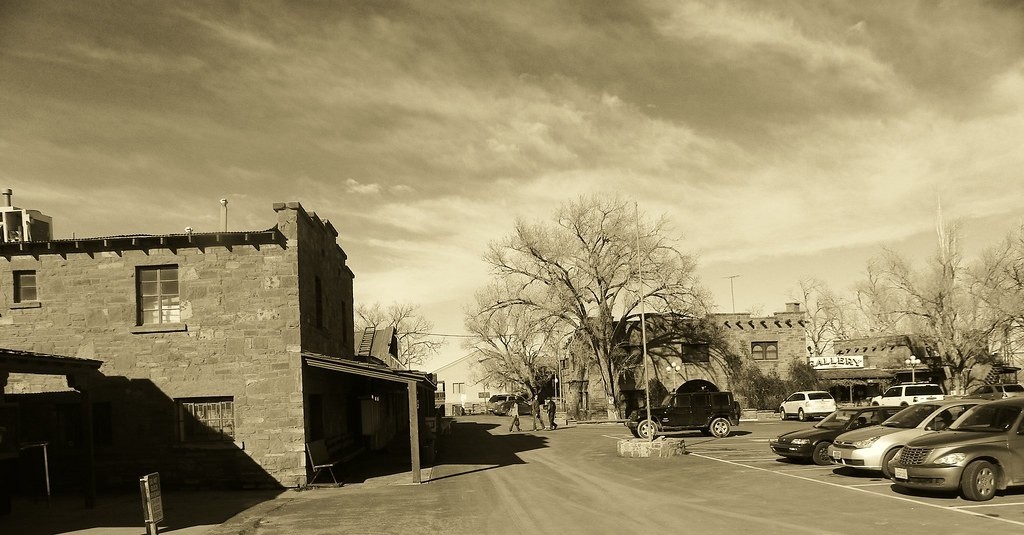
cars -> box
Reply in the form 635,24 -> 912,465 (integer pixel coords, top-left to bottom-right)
887,396 -> 1024,502
770,405 -> 908,466
828,399 -> 993,480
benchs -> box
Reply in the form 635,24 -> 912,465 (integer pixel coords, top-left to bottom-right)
305,432 -> 365,486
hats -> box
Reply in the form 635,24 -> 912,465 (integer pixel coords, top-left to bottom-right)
545,397 -> 551,400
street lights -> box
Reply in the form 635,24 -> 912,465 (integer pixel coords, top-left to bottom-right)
904,355 -> 922,383
666,362 -> 681,393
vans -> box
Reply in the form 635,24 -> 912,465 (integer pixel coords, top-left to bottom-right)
961,384 -> 1024,400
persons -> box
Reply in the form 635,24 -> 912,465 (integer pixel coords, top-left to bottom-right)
532,395 -> 558,431
508,399 -> 522,432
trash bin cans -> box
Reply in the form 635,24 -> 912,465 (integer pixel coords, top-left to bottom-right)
451,404 -> 462,417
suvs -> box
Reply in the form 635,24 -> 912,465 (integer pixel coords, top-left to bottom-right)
487,394 -> 534,416
778,391 -> 837,421
870,383 -> 946,406
624,391 -> 741,438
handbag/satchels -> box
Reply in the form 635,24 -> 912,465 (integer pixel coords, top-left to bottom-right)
514,420 -> 519,427
